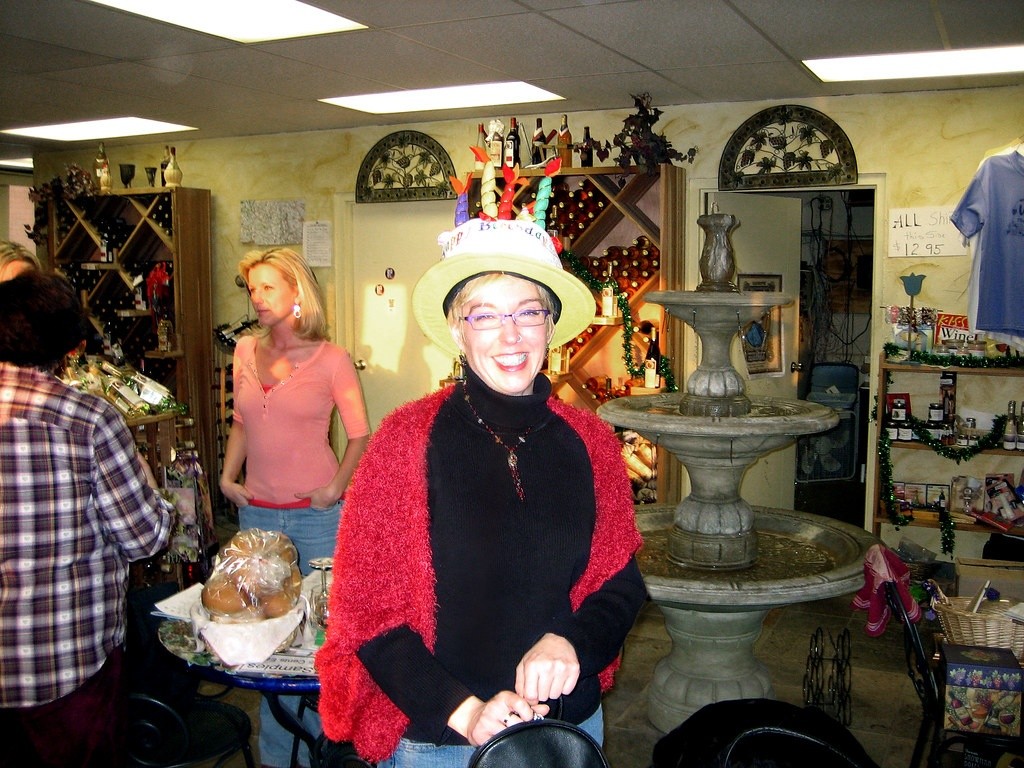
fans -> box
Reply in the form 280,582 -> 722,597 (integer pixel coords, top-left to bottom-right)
794,409 -> 859,483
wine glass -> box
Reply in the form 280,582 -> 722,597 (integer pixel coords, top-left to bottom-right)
145,167 -> 157,187
119,164 -> 135,188
308,557 -> 333,632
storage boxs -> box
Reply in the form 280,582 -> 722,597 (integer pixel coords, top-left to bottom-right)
955,558 -> 1024,602
939,643 -> 1024,737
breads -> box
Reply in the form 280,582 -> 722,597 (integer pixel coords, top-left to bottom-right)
201,529 -> 301,624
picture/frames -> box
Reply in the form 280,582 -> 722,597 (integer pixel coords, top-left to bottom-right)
735,273 -> 786,378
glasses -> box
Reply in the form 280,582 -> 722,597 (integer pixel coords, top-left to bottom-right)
452,307 -> 551,330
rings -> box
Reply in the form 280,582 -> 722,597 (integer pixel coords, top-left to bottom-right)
504,712 -> 515,727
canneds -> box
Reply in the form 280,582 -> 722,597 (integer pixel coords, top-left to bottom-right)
931,340 -> 986,359
884,402 -> 980,446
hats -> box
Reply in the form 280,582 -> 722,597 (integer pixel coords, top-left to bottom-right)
411,220 -> 596,354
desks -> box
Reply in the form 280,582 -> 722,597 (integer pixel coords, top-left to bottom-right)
160,617 -> 325,768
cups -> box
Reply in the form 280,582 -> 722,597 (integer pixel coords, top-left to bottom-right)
309,584 -> 332,629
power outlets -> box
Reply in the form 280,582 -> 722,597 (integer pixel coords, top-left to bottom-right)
817,195 -> 831,210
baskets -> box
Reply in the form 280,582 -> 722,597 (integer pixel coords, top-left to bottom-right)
930,594 -> 1024,660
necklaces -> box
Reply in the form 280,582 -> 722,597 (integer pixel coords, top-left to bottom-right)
463,385 -> 540,500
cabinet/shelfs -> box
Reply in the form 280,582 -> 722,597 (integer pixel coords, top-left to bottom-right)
46,184 -> 222,517
872,350 -> 1024,538
464,162 -> 685,503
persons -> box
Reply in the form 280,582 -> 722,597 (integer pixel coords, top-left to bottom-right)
219,247 -> 372,768
0,271 -> 177,768
314,217 -> 646,768
0,240 -> 104,358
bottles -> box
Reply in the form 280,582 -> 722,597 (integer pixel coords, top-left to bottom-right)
163,147 -> 183,187
93,141 -> 112,190
1002,400 -> 1024,451
474,117 -> 520,170
161,145 -> 171,187
939,491 -> 946,511
931,338 -> 987,358
55,193 -> 173,355
63,360 -> 176,420
468,179 -> 661,318
211,316 -> 259,484
532,118 -> 546,164
581,126 -> 593,167
555,114 -> 572,168
548,319 -> 660,406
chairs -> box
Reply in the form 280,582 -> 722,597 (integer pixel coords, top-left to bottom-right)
120,581 -> 255,768
465,718 -> 611,768
650,699 -> 881,768
850,542 -> 1024,768
